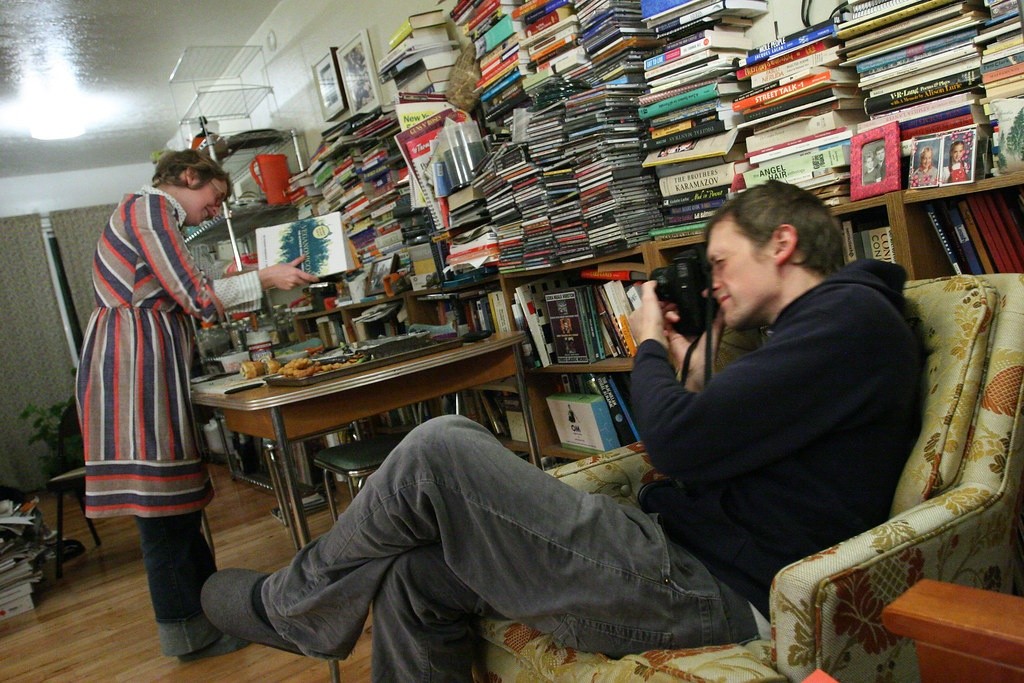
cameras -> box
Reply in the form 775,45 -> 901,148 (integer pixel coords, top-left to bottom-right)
648,249 -> 721,339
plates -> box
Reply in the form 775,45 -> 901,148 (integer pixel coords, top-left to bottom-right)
275,346 -> 323,363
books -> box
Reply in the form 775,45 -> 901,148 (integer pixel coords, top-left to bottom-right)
0,496 -> 48,622
286,0 -> 1024,448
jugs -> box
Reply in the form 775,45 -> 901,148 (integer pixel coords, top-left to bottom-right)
250,154 -> 292,205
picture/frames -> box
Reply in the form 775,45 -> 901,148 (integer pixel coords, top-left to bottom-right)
365,253 -> 400,297
908,129 -> 977,188
310,47 -> 350,123
335,28 -> 383,116
848,121 -> 901,204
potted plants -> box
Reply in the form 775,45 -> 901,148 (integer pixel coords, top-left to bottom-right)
20,396 -> 84,493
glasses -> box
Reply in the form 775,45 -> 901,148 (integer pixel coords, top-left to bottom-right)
209,181 -> 226,207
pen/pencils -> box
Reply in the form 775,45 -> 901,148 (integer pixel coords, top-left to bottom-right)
225,383 -> 264,395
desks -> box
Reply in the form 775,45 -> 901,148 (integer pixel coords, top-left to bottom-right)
191,330 -> 545,683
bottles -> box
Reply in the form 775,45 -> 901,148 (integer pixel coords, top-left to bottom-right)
196,321 -> 233,359
230,316 -> 255,353
246,330 -> 274,365
273,305 -> 299,345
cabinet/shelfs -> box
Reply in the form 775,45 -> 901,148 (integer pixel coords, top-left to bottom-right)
292,171 -> 1024,501
168,46 -> 364,491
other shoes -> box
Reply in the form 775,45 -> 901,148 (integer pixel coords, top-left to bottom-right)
201,567 -> 306,656
177,633 -> 253,662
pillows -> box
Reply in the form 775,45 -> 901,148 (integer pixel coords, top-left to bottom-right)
989,97 -> 1024,176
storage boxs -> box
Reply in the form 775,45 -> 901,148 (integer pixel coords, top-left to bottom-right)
546,392 -> 621,454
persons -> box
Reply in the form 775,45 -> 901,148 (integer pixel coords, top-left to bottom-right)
862,145 -> 886,184
913,146 -> 938,187
75,150 -> 320,662
201,179 -> 924,683
944,141 -> 970,183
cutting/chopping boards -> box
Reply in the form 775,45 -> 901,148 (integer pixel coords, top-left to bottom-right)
192,374 -> 277,394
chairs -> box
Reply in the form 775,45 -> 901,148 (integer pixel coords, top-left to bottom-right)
313,393 -> 458,524
46,395 -> 102,580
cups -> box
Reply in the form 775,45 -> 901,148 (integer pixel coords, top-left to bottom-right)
348,282 -> 368,304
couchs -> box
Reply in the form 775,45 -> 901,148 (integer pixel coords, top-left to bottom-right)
467,270 -> 1024,683
